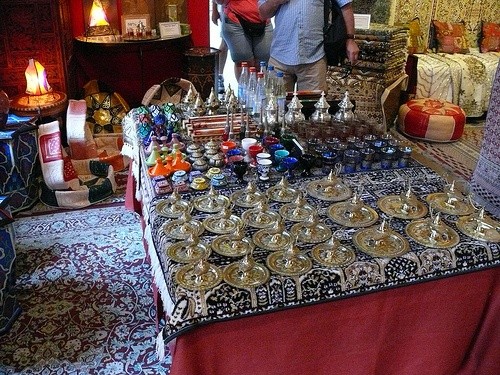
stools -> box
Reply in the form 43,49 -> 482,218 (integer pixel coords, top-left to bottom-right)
15,93 -> 68,124
395,100 -> 467,142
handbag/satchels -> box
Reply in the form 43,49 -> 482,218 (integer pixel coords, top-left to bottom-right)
322,0 -> 348,67
229,8 -> 267,38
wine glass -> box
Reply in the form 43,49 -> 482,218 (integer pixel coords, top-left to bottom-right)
221,136 -> 315,185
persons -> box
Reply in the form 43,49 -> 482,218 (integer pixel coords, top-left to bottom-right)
258,0 -> 359,98
211,0 -> 228,94
216,0 -> 276,83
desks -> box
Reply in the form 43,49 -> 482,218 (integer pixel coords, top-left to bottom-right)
125,93 -> 500,375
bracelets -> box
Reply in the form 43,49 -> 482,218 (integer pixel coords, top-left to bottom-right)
347,34 -> 356,39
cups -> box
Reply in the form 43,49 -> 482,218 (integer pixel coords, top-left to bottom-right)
297,120 -> 413,174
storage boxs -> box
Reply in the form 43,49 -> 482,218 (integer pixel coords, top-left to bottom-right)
182,45 -> 223,100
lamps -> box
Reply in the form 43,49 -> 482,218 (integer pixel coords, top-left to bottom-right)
87,1 -> 115,39
22,57 -> 55,103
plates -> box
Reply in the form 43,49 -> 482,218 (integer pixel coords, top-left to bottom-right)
157,179 -> 500,289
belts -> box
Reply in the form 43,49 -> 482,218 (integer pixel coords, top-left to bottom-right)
220,17 -> 234,24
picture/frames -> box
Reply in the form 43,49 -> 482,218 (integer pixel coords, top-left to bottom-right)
121,13 -> 150,38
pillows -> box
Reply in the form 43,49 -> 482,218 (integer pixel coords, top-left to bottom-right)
432,18 -> 470,54
480,18 -> 500,53
395,18 -> 426,55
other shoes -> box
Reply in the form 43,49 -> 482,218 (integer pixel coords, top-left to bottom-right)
217,74 -> 225,94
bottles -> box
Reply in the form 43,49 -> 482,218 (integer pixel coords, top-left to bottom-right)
259,61 -> 267,85
265,65 -> 277,106
238,61 -> 249,111
253,72 -> 266,129
272,71 -> 286,118
246,67 -> 257,116
